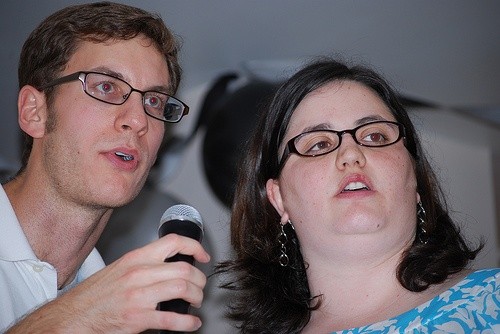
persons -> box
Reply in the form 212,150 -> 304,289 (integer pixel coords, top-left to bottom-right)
0,2 -> 209,334
205,60 -> 500,334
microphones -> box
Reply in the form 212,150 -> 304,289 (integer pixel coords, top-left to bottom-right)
160,205 -> 204,334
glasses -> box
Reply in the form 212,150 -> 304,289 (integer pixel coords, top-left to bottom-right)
36,70 -> 189,124
275,121 -> 406,181
